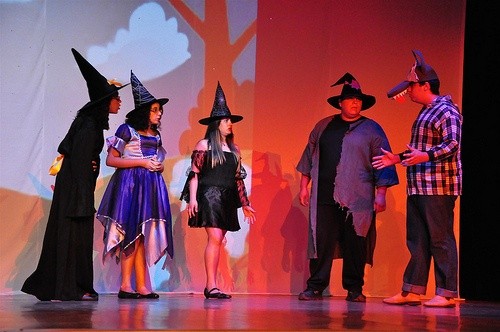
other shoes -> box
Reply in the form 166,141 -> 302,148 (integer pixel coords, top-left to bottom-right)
117,290 -> 141,298
204,287 -> 221,298
383,291 -> 421,306
423,294 -> 455,308
219,289 -> 231,299
141,292 -> 159,298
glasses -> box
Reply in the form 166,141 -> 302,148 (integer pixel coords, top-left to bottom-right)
151,108 -> 162,113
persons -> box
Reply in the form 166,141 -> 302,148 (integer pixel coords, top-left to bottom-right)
20,78 -> 122,303
294,84 -> 399,303
180,103 -> 256,300
371,64 -> 463,308
97,94 -> 173,299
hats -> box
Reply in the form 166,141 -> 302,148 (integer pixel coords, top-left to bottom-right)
327,73 -> 376,111
126,69 -> 169,118
199,81 -> 243,125
71,47 -> 131,110
387,48 -> 439,100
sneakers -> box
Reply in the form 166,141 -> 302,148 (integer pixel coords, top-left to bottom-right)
346,294 -> 366,302
298,288 -> 322,300
